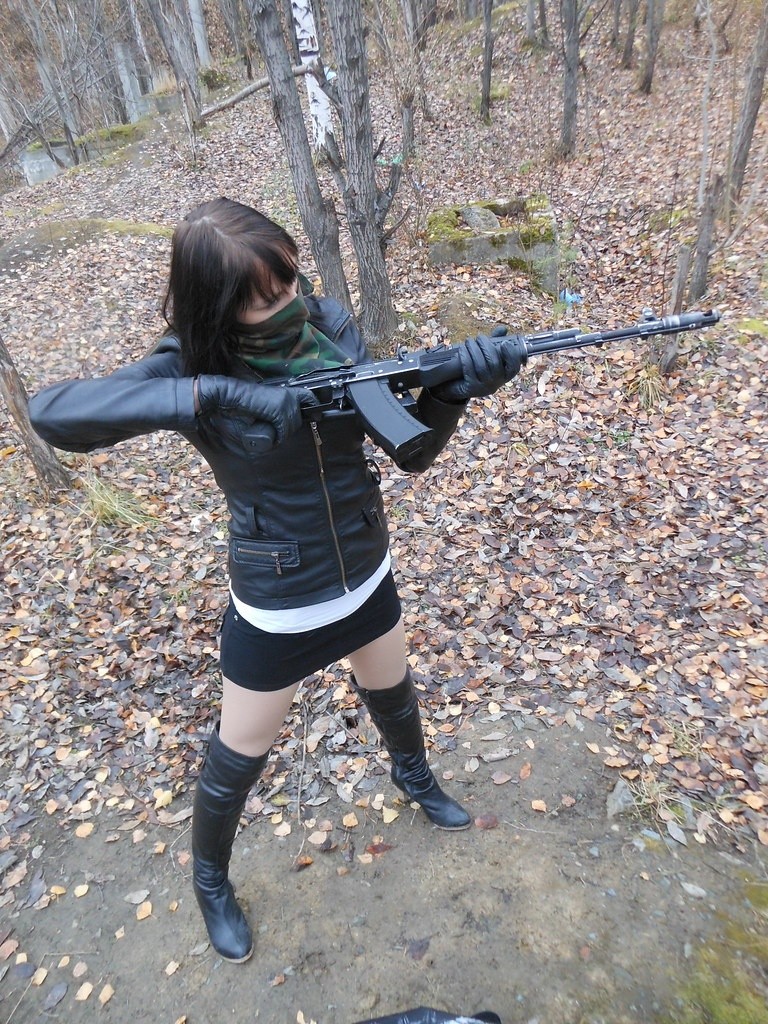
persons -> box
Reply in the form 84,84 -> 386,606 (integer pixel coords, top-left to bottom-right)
26,194 -> 527,967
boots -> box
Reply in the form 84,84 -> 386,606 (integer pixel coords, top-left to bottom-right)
349,664 -> 471,831
192,720 -> 269,963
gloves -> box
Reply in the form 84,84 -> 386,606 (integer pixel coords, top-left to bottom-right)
197,374 -> 318,447
432,327 -> 521,405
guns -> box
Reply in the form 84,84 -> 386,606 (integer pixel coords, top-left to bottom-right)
215,305 -> 725,469
